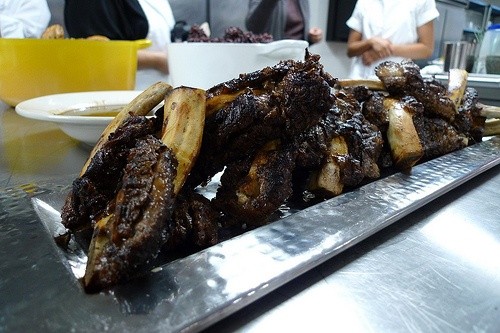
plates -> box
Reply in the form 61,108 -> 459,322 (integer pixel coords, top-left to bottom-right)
16,89 -> 172,145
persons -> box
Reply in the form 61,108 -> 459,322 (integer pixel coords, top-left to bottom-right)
63,0 -> 175,92
246,0 -> 324,46
346,0 -> 439,80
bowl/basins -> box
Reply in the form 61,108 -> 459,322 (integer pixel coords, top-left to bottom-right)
169,39 -> 312,91
0,38 -> 152,105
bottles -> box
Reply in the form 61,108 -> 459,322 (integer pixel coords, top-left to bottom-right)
480,24 -> 500,77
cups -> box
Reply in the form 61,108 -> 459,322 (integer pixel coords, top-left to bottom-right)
444,40 -> 468,73
459,29 -> 479,73
